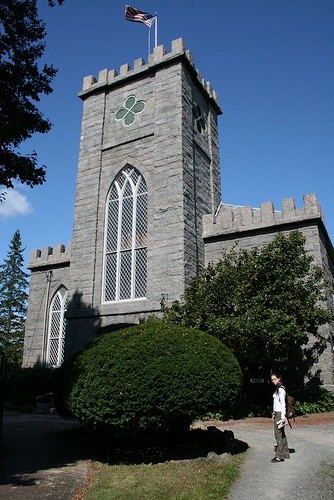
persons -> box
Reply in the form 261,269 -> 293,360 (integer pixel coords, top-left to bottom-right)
270,373 -> 294,462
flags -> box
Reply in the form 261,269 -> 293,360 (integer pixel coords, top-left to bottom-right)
124,5 -> 154,28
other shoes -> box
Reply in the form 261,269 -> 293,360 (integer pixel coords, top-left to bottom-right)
271,457 -> 284,463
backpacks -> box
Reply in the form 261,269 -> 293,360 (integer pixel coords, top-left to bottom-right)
286,394 -> 295,419
